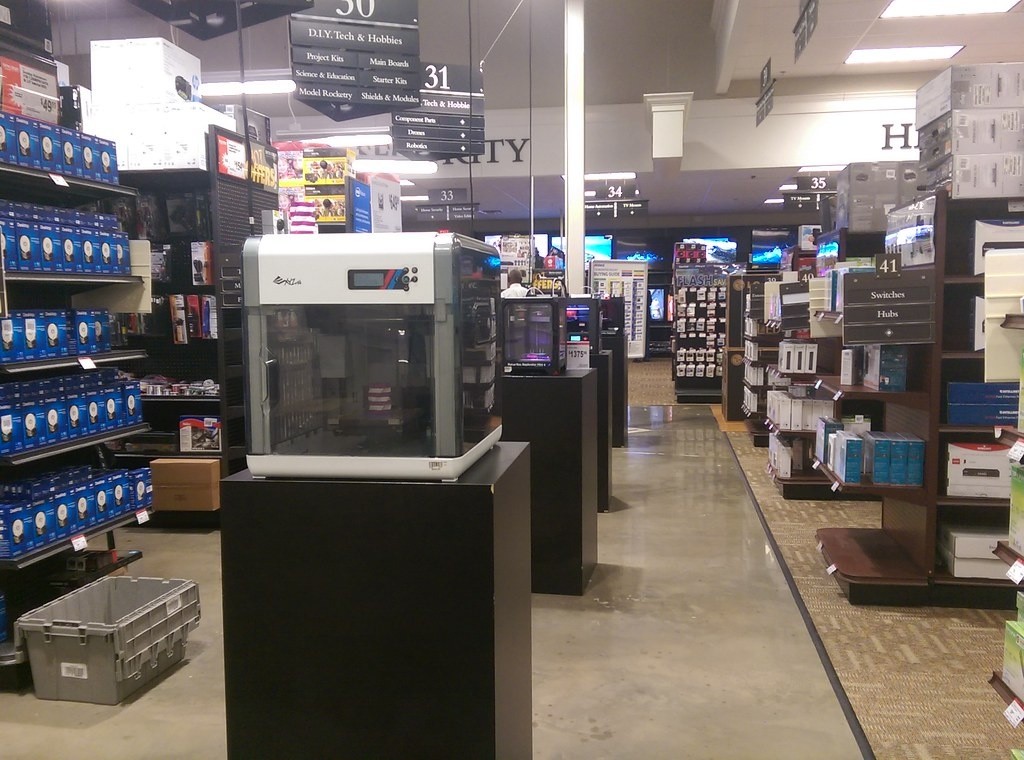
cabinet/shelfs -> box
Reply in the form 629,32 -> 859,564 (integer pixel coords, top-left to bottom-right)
102,123 -> 253,477
987,310 -> 1024,728
0,161 -> 158,692
277,175 -> 371,234
807,185 -> 1024,610
741,270 -> 783,448
765,227 -> 888,501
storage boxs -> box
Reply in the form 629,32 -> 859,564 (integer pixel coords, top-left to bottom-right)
15,573 -> 202,706
0,101 -> 236,560
277,146 -> 358,223
740,58 -> 1024,704
90,35 -> 200,114
